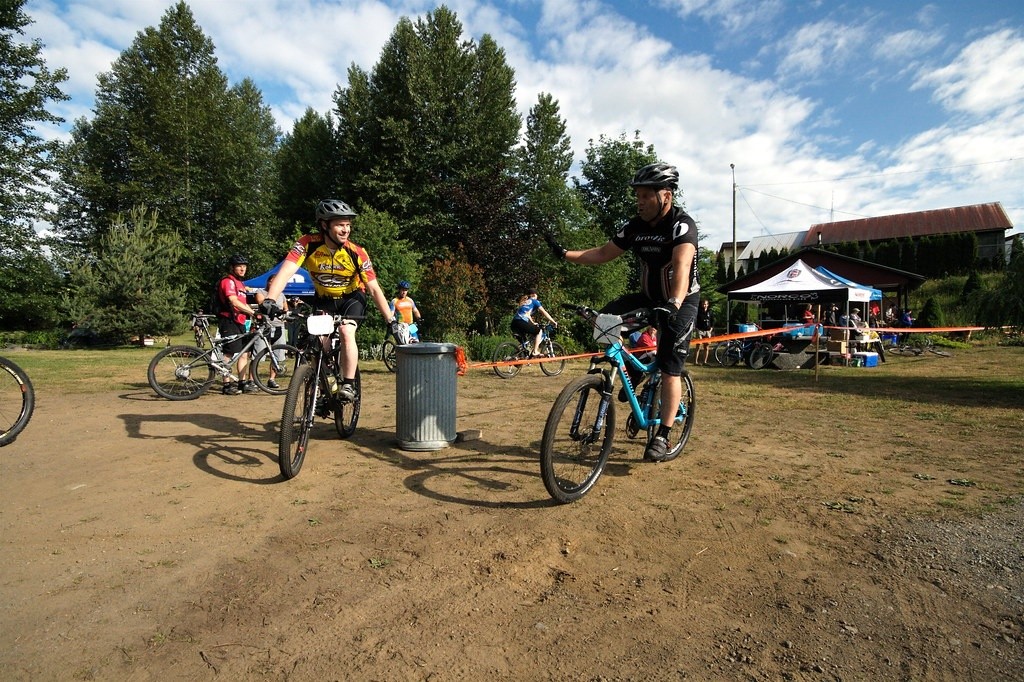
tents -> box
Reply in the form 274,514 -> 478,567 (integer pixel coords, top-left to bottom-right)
726,258 -> 884,367
241,260 -> 315,295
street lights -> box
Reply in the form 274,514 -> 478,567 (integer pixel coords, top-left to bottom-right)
730,163 -> 736,280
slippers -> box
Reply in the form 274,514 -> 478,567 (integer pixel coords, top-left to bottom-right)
531,351 -> 544,357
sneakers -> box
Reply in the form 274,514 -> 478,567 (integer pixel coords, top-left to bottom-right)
643,434 -> 671,462
339,384 -> 355,403
618,372 -> 646,402
223,386 -> 242,395
267,380 -> 279,388
239,383 -> 254,392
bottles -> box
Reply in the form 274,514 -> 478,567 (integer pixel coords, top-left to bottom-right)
323,364 -> 339,395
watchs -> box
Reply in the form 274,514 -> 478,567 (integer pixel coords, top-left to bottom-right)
668,297 -> 681,306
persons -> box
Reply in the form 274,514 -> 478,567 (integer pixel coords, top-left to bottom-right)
545,162 -> 700,463
247,274 -> 280,388
887,307 -> 898,321
191,309 -> 209,345
839,307 -> 850,326
802,303 -> 814,323
850,308 -> 861,323
215,254 -> 257,395
694,298 -> 714,366
901,309 -> 916,343
860,321 -> 885,363
511,289 -> 557,357
389,280 -> 421,344
286,296 -> 313,358
256,199 -> 398,416
825,303 -> 836,326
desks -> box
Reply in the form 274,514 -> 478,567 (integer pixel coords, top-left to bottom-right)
771,336 -> 831,342
847,339 -> 880,351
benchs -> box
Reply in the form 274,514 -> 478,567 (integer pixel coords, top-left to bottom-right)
802,349 -> 846,369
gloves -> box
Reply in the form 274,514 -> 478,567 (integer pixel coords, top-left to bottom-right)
255,312 -> 265,323
259,299 -> 276,321
648,302 -> 678,331
543,233 -> 567,261
385,321 -> 401,344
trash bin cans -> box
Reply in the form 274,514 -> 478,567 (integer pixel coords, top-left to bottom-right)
395,342 -> 458,451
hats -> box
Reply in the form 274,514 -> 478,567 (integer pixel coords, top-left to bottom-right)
527,288 -> 541,297
854,308 -> 860,311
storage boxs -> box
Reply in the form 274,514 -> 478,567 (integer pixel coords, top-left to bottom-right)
783,323 -> 804,336
855,335 -> 869,340
853,351 -> 879,367
827,340 -> 847,354
144,339 -> 154,346
882,335 -> 897,345
805,323 -> 823,335
739,323 -> 759,333
831,329 -> 847,340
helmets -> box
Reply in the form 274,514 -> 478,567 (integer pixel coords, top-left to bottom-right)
198,309 -> 203,312
229,255 -> 249,265
630,164 -> 679,188
399,281 -> 410,288
315,199 -> 357,225
291,296 -> 298,302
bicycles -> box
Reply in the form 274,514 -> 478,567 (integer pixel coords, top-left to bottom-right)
540,302 -> 696,504
147,312 -> 308,401
712,320 -> 773,370
889,338 -> 952,357
492,323 -> 566,379
191,325 -> 208,348
0,356 -> 35,446
259,304 -> 368,478
383,319 -> 424,373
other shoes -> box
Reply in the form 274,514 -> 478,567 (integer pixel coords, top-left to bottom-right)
695,363 -> 701,366
703,363 -> 710,366
